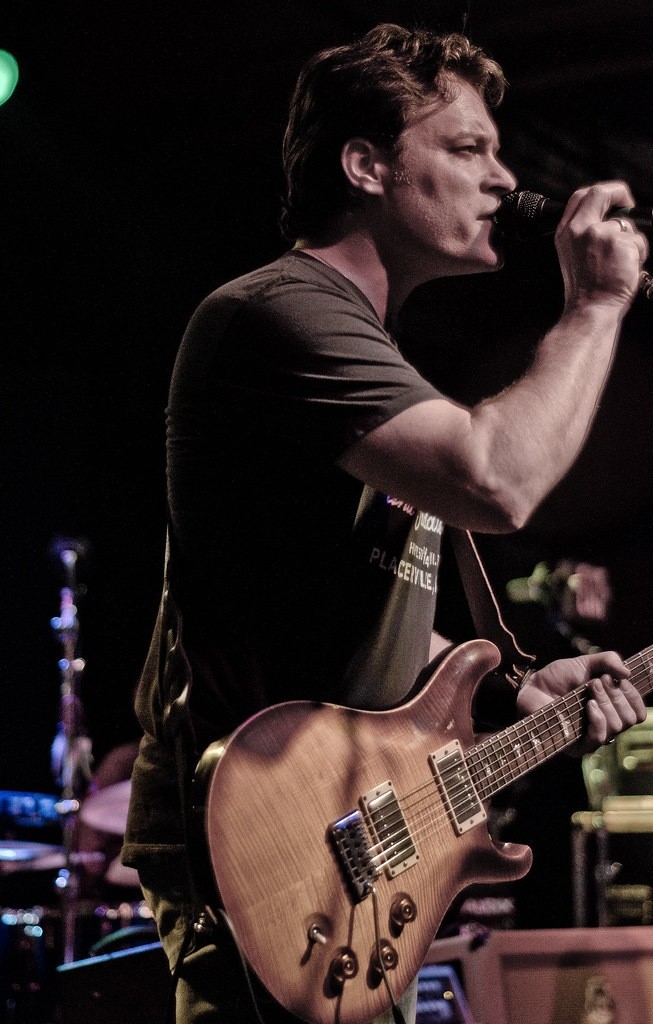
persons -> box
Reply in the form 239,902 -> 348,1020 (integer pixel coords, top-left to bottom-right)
120,22 -> 648,1024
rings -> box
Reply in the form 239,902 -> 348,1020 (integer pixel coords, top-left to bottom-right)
607,739 -> 615,743
612,218 -> 627,232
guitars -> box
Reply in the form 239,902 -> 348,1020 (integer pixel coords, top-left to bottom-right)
186,633 -> 652,1024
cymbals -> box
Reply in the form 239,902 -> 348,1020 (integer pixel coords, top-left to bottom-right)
78,776 -> 131,836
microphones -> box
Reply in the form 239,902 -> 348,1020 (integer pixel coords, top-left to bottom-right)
496,191 -> 653,239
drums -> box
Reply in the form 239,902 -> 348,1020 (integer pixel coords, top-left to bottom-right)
0,791 -> 103,926
